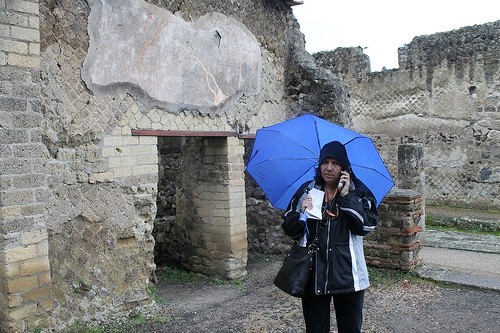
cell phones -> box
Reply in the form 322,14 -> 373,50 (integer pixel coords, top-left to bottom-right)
337,170 -> 347,189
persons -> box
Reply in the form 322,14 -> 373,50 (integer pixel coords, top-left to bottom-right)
245,113 -> 395,212
280,140 -> 380,333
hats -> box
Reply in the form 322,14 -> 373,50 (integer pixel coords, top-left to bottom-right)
319,140 -> 350,168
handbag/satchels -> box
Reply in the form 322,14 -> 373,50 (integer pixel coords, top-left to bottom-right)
273,243 -> 314,299
